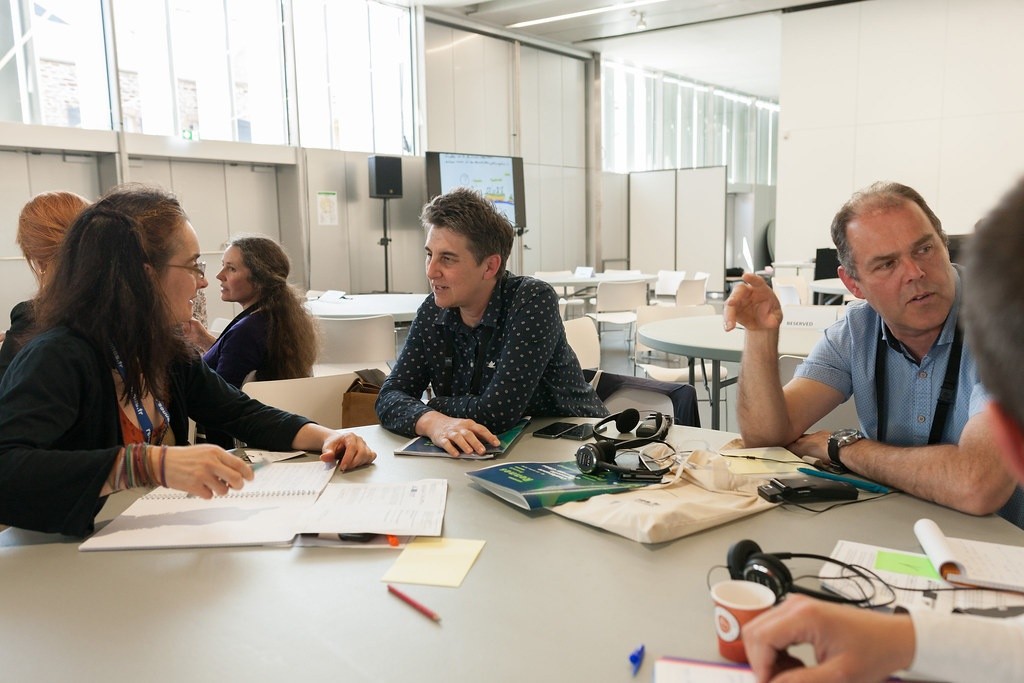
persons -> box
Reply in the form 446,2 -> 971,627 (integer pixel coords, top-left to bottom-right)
0,193 -> 91,385
724,181 -> 1024,528
375,189 -> 611,458
182,236 -> 317,451
0,188 -> 377,535
741,174 -> 1024,683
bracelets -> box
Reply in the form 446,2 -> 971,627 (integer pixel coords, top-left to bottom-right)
108,443 -> 168,491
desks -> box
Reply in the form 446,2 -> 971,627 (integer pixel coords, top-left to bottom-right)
812,278 -> 855,306
0,417 -> 1024,683
542,276 -> 659,305
303,293 -> 431,322
638,315 -> 826,430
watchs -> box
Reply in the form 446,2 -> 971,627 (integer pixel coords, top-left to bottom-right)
828,428 -> 865,464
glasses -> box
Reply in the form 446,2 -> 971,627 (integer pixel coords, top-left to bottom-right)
163,262 -> 206,278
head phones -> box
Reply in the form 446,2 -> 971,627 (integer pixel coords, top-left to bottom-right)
726,539 -> 876,606
592,408 -> 669,444
575,439 -> 676,475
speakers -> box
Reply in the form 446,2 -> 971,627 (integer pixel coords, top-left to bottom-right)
367,155 -> 403,198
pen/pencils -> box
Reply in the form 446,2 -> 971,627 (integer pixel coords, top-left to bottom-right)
388,585 -> 440,622
797,468 -> 888,494
386,536 -> 400,548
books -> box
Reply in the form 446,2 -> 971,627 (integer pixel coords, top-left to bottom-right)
914,519 -> 1024,593
79,460 -> 339,551
393,416 -> 531,460
464,461 -> 647,513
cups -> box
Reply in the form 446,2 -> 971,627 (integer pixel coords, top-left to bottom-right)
710,580 -> 777,663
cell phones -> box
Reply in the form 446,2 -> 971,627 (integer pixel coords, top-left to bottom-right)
533,421 -> 578,439
562,423 -> 607,440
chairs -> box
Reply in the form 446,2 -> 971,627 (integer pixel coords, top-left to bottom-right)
210,260 -> 867,435
